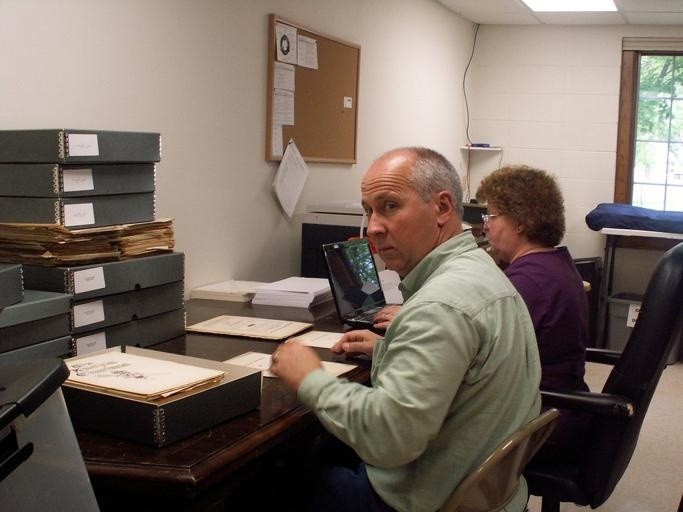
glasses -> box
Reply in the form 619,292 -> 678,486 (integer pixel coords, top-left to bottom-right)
481,213 -> 511,224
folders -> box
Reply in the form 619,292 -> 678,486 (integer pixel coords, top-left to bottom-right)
0,129 -> 186,359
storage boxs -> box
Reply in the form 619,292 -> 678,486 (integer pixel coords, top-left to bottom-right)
605,284 -> 683,368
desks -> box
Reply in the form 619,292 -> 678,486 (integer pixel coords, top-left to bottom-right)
73,290 -> 387,512
593,227 -> 683,362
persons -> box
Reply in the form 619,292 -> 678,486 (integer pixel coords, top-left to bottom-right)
244,148 -> 543,511
373,164 -> 592,434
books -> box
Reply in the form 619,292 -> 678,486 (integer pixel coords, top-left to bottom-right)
187,274 -> 335,312
61,350 -> 225,408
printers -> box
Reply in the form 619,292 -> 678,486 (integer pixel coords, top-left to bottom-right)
301,203 -> 368,278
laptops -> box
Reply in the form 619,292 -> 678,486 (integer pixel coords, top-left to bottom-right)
322,238 -> 403,337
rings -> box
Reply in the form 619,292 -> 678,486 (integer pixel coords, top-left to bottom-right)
273,350 -> 279,362
384,315 -> 388,320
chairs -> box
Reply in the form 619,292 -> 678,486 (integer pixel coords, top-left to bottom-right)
520,234 -> 683,512
440,402 -> 561,512
